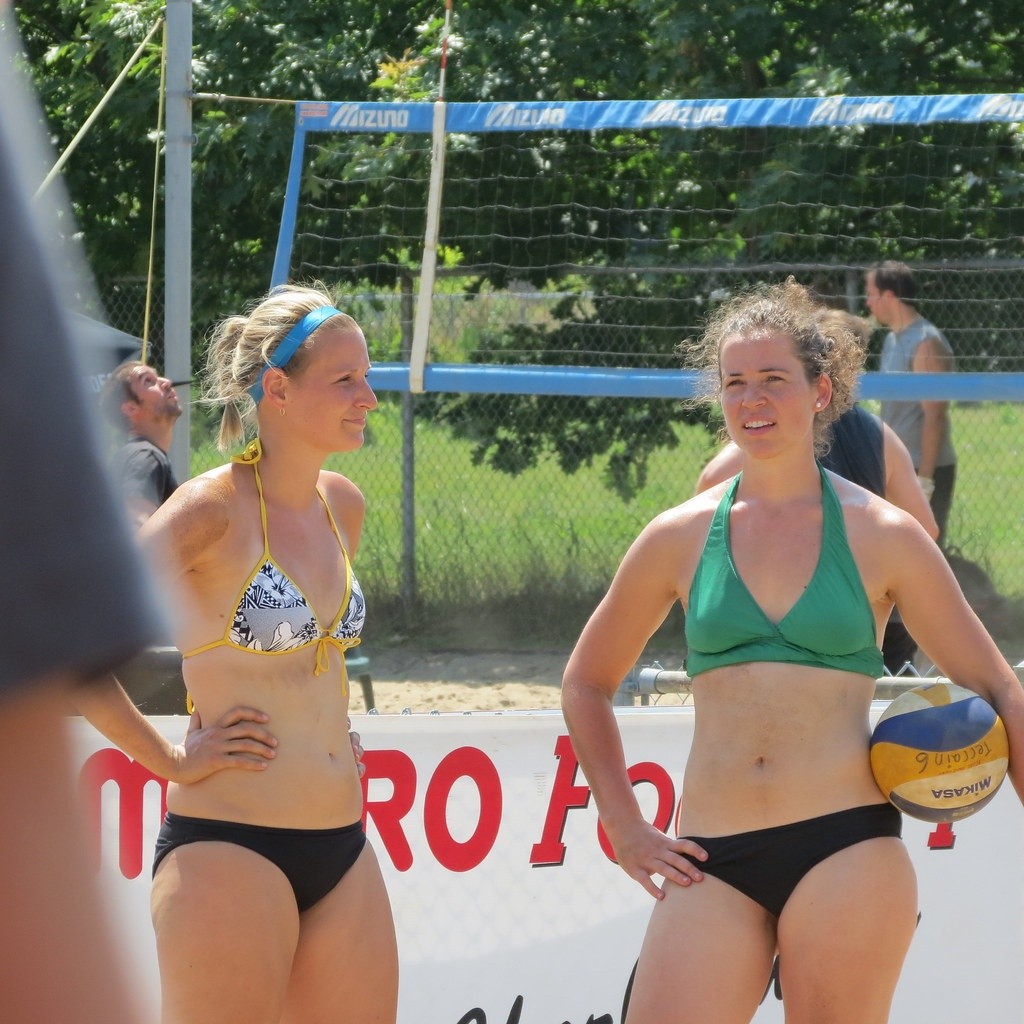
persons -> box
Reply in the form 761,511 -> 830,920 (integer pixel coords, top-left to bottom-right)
559,279 -> 1023,1023
76,275 -> 402,1023
693,309 -> 941,542
100,342 -> 192,719
864,258 -> 1022,679
2,1 -> 179,1023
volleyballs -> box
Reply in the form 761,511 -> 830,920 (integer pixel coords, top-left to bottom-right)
869,679 -> 1011,825
